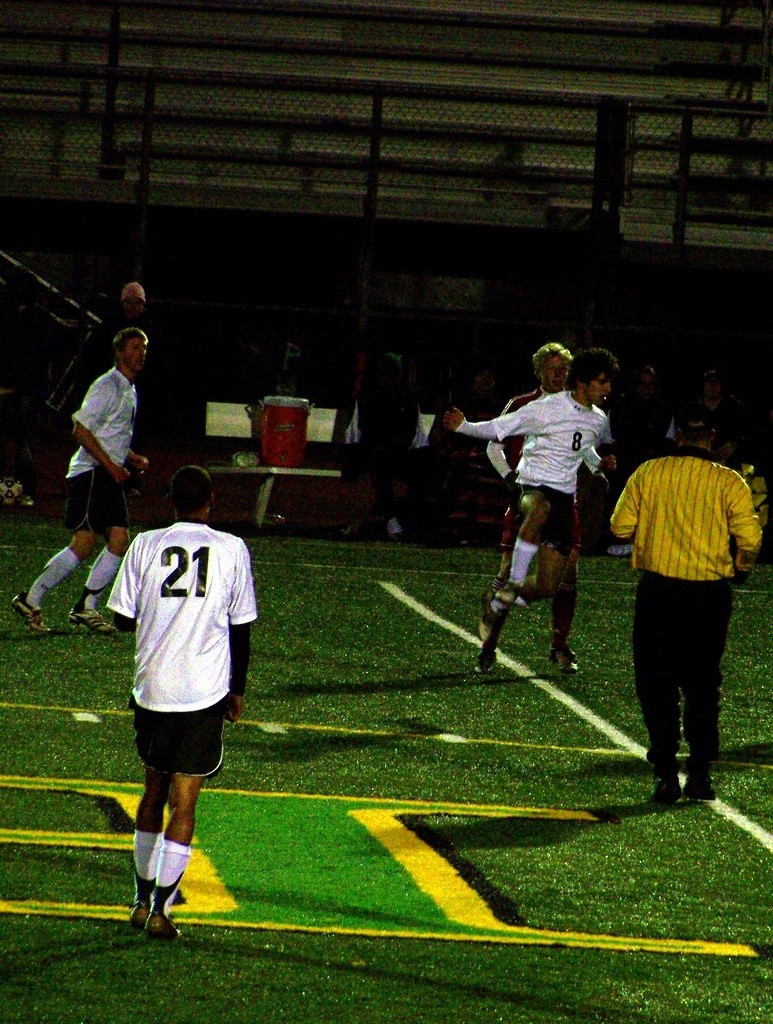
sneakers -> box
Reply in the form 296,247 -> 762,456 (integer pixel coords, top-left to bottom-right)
10,590 -> 52,635
67,604 -> 116,634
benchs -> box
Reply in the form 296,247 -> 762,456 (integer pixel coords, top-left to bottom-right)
205,402 -> 436,527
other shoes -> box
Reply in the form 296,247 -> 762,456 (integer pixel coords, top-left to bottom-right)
546,640 -> 578,673
649,780 -> 683,805
128,906 -> 148,930
479,589 -> 497,621
683,780 -> 717,799
147,911 -> 181,937
479,618 -> 492,639
475,649 -> 497,675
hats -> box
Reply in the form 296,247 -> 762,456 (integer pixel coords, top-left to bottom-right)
673,402 -> 715,431
702,370 -> 719,380
637,367 -> 656,377
121,282 -> 147,303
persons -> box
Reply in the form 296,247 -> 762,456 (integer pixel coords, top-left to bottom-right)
443,348 -> 620,640
474,342 -> 578,675
433,362 -> 500,478
609,404 -> 763,804
351,353 -> 418,539
12,328 -> 149,632
106,466 -> 258,938
121,282 -> 146,317
610,367 -> 750,485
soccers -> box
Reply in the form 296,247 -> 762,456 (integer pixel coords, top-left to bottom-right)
0,474 -> 24,508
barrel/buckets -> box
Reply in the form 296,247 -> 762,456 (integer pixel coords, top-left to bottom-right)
260,396 -> 313,469
